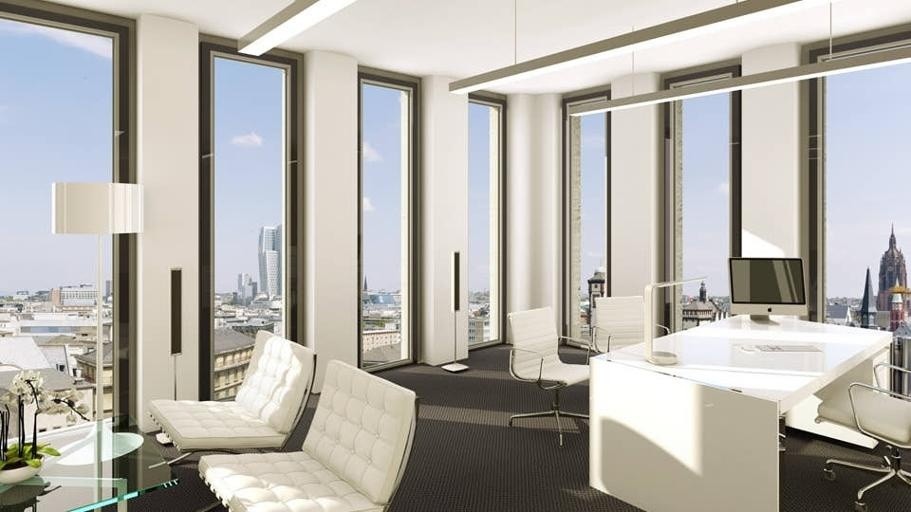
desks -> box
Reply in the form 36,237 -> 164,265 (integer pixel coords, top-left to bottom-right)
591,310 -> 899,512
0,410 -> 185,512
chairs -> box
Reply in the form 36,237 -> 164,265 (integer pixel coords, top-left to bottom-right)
500,301 -> 590,450
813,357 -> 911,512
144,324 -> 322,475
584,290 -> 671,357
194,355 -> 423,511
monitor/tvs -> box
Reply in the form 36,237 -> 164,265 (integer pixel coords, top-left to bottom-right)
728,257 -> 807,326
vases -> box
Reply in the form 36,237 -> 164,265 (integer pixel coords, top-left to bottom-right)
0,433 -> 62,489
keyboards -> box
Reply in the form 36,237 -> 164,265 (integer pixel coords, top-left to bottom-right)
741,343 -> 822,353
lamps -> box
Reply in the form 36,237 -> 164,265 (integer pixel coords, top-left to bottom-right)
445,2 -> 821,98
153,263 -> 187,446
232,0 -> 366,64
565,1 -> 911,120
441,249 -> 473,375
640,274 -> 712,367
48,178 -> 148,511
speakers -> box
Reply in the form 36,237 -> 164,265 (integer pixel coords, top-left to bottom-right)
452,252 -> 459,311
170,269 -> 181,354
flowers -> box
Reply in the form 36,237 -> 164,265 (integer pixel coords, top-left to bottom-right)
0,366 -> 61,472
